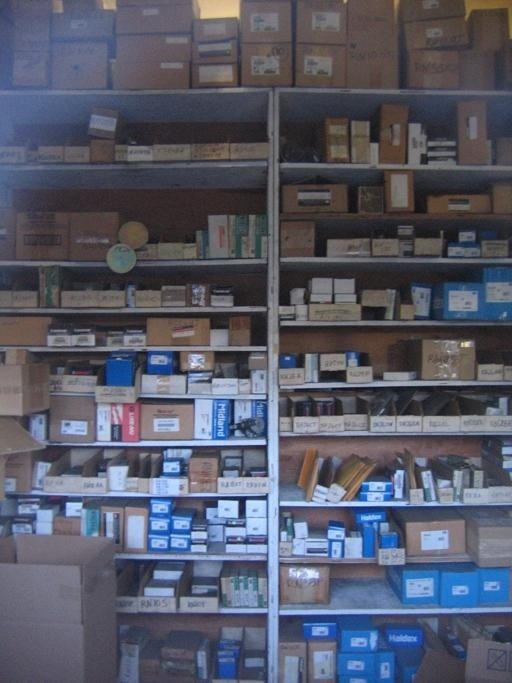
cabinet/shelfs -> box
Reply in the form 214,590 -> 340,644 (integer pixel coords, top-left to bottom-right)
2,88 -> 274,682
274,89 -> 512,681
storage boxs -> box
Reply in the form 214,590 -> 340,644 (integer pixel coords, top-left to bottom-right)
3,1 -> 511,88
